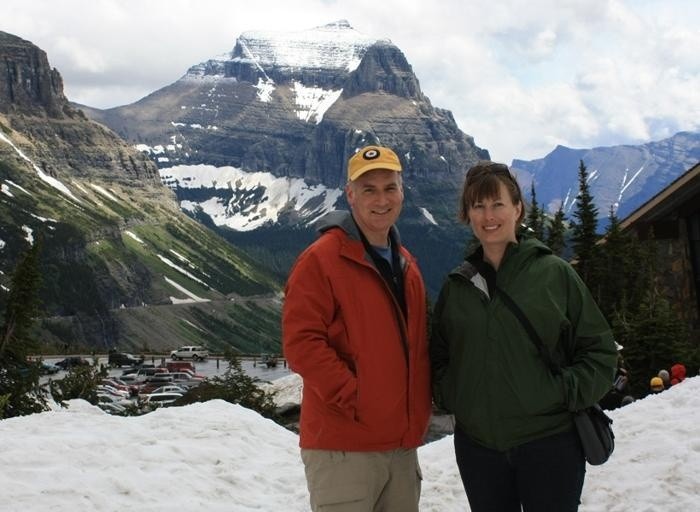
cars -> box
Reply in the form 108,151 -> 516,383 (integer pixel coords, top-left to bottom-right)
95,346 -> 208,415
42,357 -> 89,374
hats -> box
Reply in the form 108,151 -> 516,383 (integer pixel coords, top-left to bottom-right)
649,364 -> 686,388
614,340 -> 624,351
345,143 -> 404,181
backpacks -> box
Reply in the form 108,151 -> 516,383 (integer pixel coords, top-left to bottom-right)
612,356 -> 629,392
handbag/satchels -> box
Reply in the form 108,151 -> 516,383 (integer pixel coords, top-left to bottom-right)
577,397 -> 616,466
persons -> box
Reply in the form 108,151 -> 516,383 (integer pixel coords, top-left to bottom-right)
429,158 -> 621,512
279,145 -> 435,512
668,363 -> 688,386
649,377 -> 666,395
622,394 -> 634,408
658,369 -> 673,391
